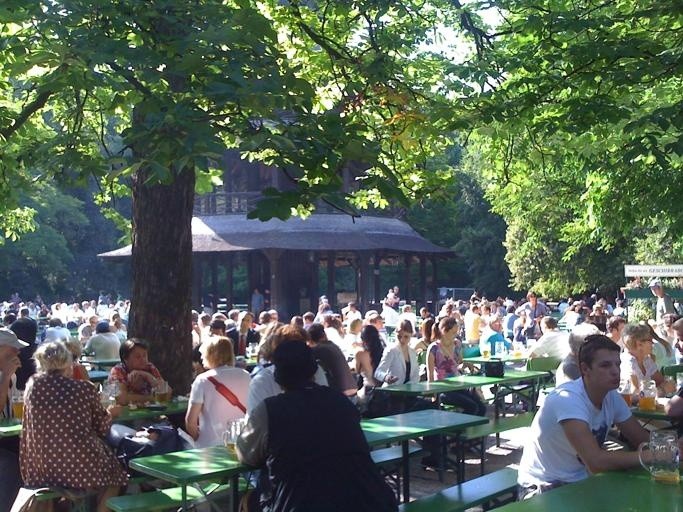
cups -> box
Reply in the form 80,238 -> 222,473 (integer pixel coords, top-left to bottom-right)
637,379 -> 657,411
476,339 -> 536,357
637,429 -> 681,486
150,380 -> 169,405
222,417 -> 245,454
10,393 -> 25,423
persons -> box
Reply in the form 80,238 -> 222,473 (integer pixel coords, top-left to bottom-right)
21,343 -> 119,512
184,337 -> 252,447
515,336 -> 683,511
1,278 -> 682,420
232,339 -> 400,512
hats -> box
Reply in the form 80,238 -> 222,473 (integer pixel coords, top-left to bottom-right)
0,327 -> 30,349
648,279 -> 662,288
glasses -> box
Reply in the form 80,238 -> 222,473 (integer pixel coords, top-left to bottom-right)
640,335 -> 652,343
583,335 -> 604,344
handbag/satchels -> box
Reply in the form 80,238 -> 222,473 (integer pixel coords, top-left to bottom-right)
485,358 -> 504,378
523,324 -> 535,336
357,385 -> 375,413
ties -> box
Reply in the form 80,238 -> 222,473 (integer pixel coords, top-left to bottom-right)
239,335 -> 246,355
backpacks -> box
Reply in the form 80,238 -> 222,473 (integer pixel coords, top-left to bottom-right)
672,299 -> 683,317
